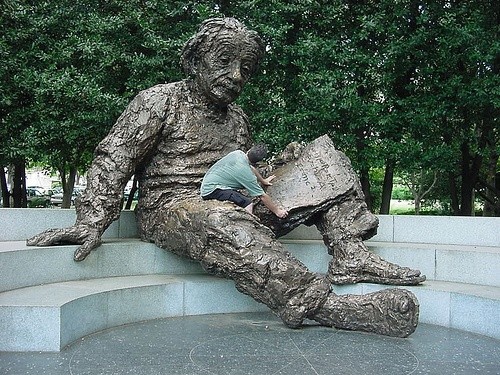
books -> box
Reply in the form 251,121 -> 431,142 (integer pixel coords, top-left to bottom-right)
255,133 -> 358,224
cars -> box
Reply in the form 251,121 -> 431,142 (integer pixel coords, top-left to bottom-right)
26,185 -> 140,208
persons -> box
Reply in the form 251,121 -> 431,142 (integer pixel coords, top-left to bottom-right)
198,145 -> 288,220
29,17 -> 430,340
